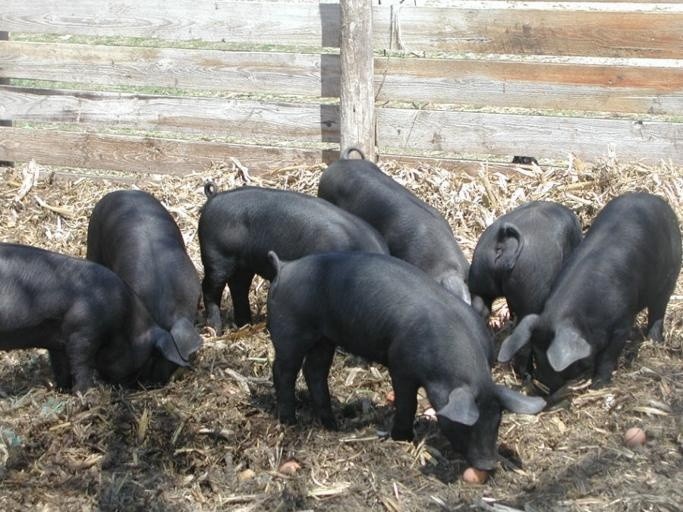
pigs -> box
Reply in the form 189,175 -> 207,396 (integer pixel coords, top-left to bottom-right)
317,146 -> 471,307
469,200 -> 583,381
86,190 -> 203,391
0,242 -> 191,397
266,250 -> 548,472
496,190 -> 683,397
197,181 -> 391,337
